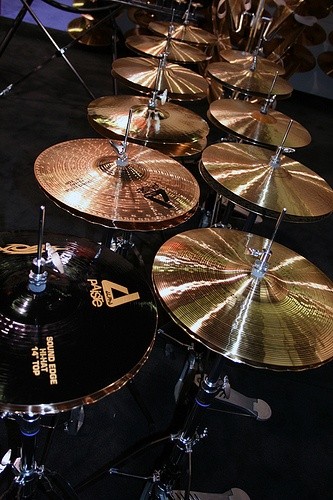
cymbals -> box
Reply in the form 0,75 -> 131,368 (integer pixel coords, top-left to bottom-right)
0,20 -> 333,418
